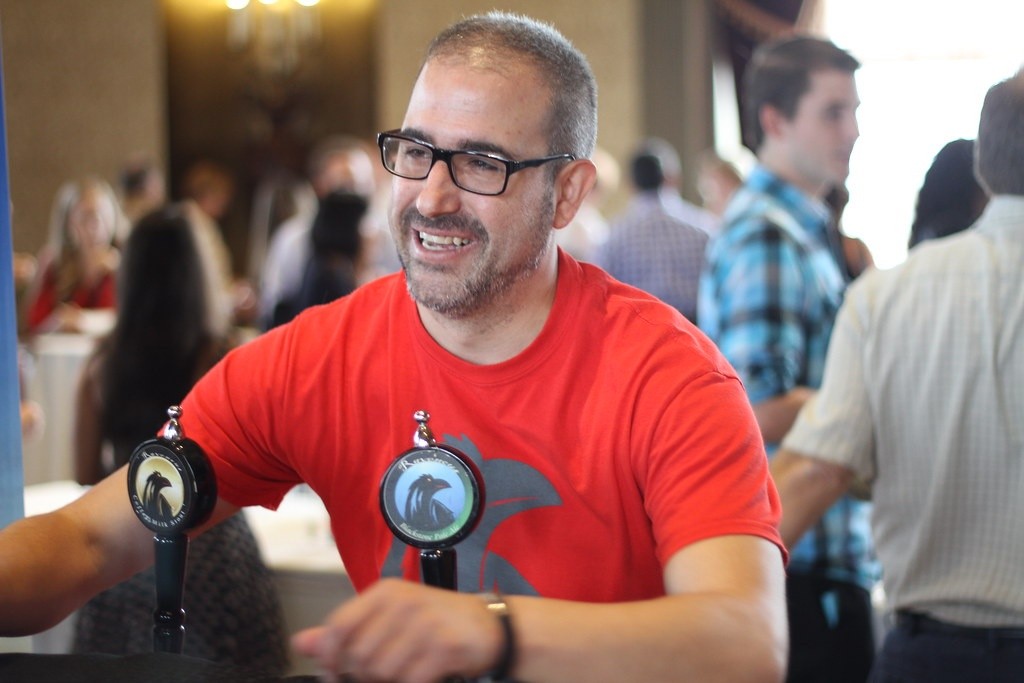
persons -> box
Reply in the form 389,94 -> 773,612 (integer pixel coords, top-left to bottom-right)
559,36 -> 1024,683
19,133 -> 406,679
0,12 -> 790,683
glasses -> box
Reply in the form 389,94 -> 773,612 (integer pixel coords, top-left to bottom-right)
376,129 -> 577,196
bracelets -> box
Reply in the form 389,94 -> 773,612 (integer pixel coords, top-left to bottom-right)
479,592 -> 518,681
